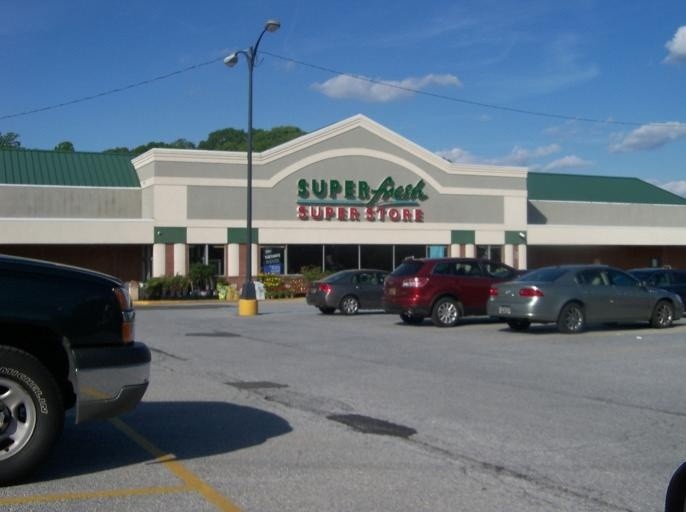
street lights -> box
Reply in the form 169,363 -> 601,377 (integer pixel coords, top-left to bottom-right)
224,20 -> 281,315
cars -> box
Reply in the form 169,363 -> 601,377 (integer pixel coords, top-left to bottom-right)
0,254 -> 151,482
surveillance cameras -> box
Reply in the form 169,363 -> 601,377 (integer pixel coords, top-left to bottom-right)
519,232 -> 525,238
